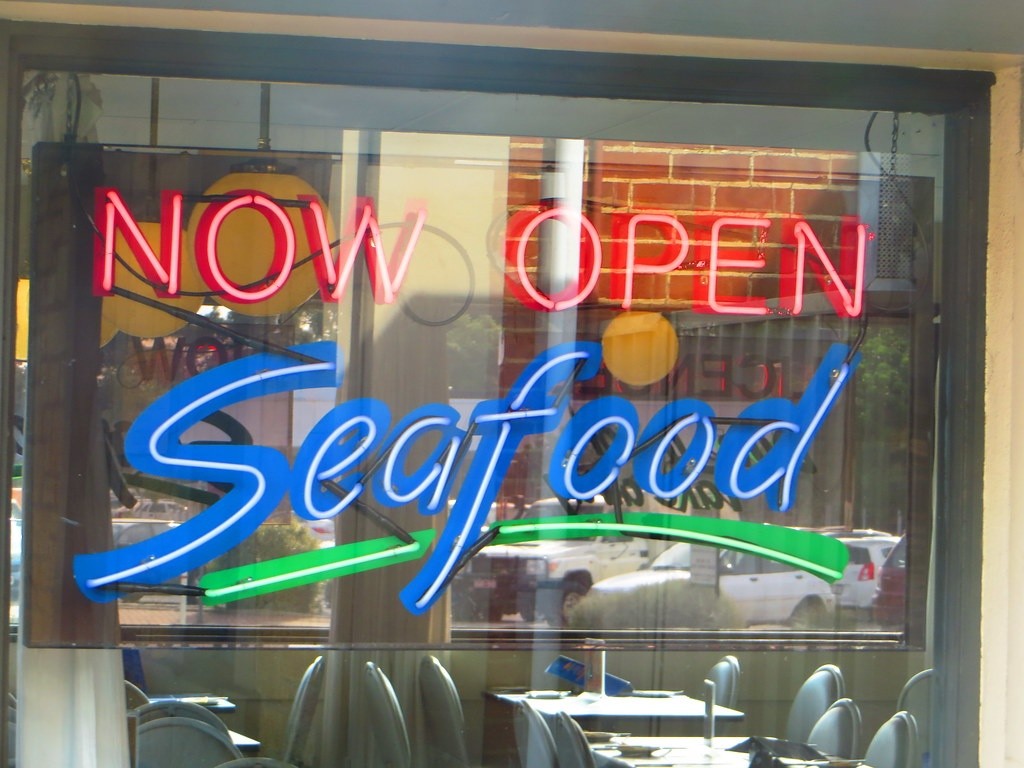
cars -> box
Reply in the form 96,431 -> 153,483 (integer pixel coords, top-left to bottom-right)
9,485 -> 335,603
445,494 -> 907,630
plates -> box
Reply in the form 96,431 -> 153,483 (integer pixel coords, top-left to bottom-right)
527,691 -> 571,700
631,690 -> 684,698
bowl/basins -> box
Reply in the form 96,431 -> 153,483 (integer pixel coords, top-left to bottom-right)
583,732 -> 632,743
609,736 -> 661,757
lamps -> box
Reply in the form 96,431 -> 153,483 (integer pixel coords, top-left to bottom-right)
602,309 -> 680,387
101,77 -> 205,339
187,84 -> 335,317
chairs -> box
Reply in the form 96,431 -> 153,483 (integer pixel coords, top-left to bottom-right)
785,664 -> 933,768
281,656 -> 326,768
124,680 -> 299,768
512,700 -> 598,768
362,661 -> 413,768
419,655 -> 472,768
707,655 -> 740,714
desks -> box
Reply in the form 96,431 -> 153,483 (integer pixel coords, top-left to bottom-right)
481,689 -> 745,720
589,737 -> 873,768
148,693 -> 236,713
228,728 -> 261,757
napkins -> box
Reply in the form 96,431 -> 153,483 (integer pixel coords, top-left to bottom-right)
726,735 -> 826,761
749,742 -> 789,768
